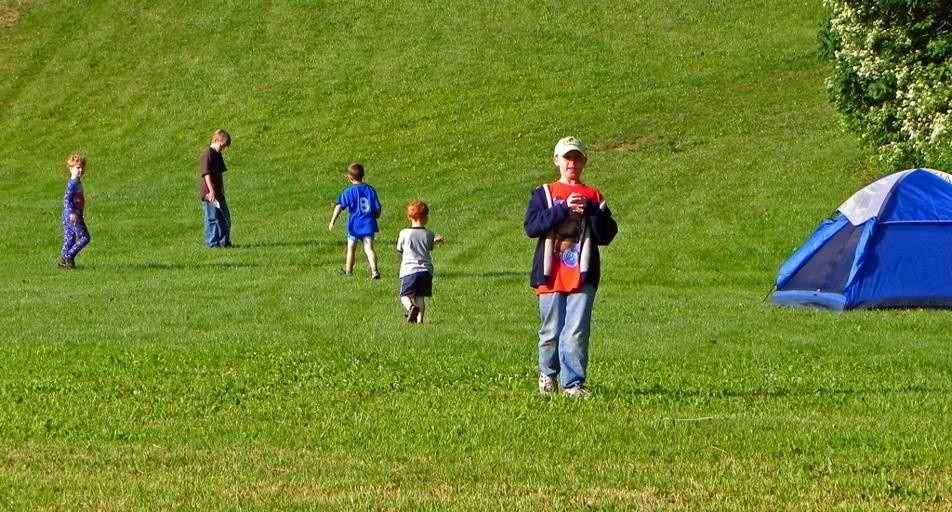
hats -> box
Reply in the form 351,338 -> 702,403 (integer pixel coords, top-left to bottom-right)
553,136 -> 587,157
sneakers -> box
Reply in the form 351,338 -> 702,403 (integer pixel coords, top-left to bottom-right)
404,305 -> 419,323
538,373 -> 558,393
372,270 -> 380,279
340,268 -> 353,277
59,257 -> 76,269
563,385 -> 589,397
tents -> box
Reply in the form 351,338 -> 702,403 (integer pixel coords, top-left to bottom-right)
763,167 -> 951,312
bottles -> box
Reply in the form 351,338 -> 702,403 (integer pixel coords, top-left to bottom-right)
205,194 -> 220,208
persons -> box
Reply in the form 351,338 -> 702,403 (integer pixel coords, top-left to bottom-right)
524,135 -> 618,400
57,154 -> 91,269
199,129 -> 234,248
328,163 -> 381,279
395,200 -> 444,322
